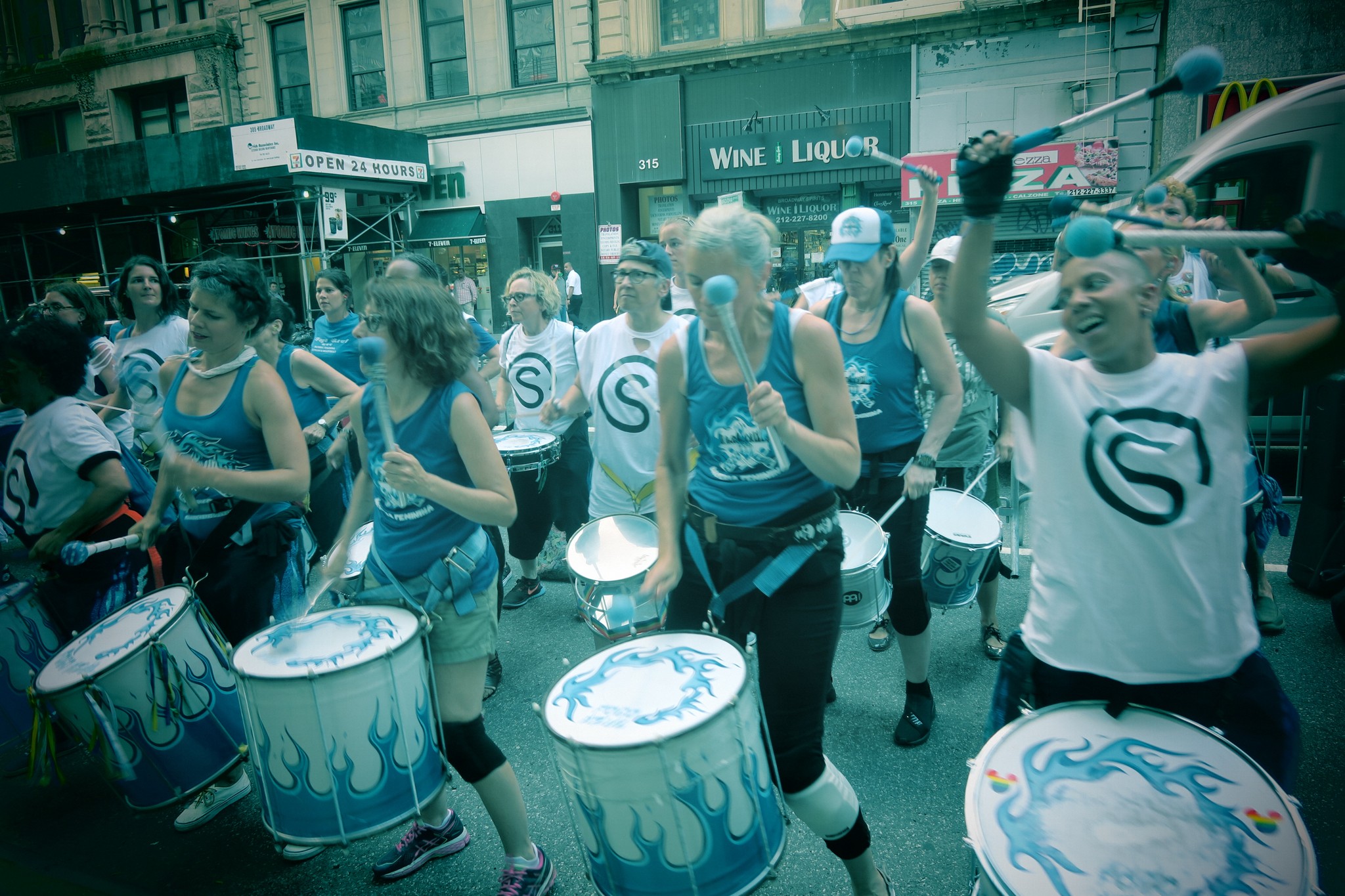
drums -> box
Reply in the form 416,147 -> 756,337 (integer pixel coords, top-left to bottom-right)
958,699 -> 1327,895
532,628 -> 791,896
835,508 -> 892,632
491,428 -> 562,474
560,512 -> 671,642
228,603 -> 450,849
33,581 -> 249,812
319,518 -> 374,597
921,486 -> 1006,615
0,581 -> 64,751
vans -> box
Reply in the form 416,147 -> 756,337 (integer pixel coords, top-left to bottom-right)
1134,75 -> 1345,494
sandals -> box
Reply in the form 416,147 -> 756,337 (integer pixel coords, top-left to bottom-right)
482,654 -> 502,700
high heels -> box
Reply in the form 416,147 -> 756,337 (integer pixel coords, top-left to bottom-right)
981,624 -> 1011,661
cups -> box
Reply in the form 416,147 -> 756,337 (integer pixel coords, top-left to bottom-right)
334,209 -> 343,230
329,217 -> 337,234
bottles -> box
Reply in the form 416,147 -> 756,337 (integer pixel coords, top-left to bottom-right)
558,272 -> 563,276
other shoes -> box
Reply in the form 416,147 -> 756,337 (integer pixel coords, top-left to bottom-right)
868,614 -> 897,651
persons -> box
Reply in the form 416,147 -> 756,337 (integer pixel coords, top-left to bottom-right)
123,253 -> 314,833
0,163 -> 1345,699
803,203 -> 966,749
1,315 -> 135,781
315,272 -> 559,895
944,125 -> 1343,814
632,204 -> 894,895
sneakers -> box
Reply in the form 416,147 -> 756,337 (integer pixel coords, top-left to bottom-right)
370,808 -> 470,880
502,563 -> 512,587
853,867 -> 897,896
895,695 -> 936,748
174,768 -> 252,831
282,842 -> 328,861
504,575 -> 547,609
494,839 -> 557,896
1250,587 -> 1287,630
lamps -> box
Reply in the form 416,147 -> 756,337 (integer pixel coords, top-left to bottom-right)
741,110 -> 762,132
814,105 -> 831,124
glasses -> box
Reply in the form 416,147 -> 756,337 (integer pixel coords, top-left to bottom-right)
610,271 -> 658,283
501,292 -> 538,303
36,302 -> 84,317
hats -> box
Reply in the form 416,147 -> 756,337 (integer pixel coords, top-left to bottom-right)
620,240 -> 672,311
921,236 -> 965,268
824,204 -> 892,268
551,264 -> 561,269
